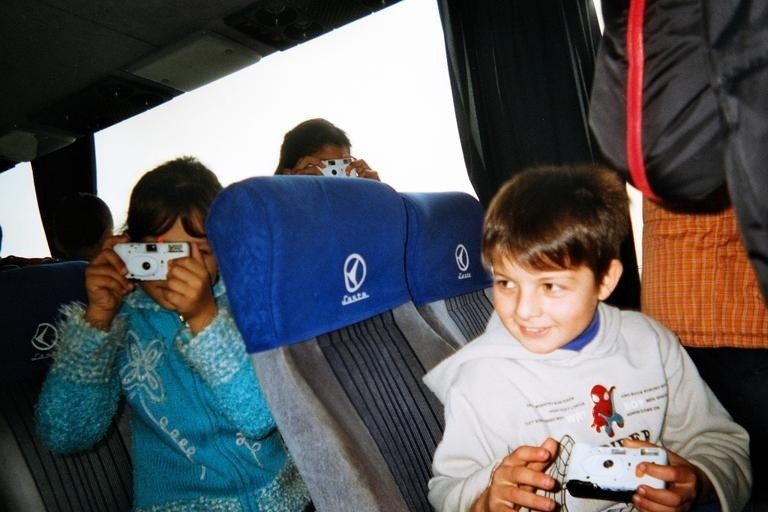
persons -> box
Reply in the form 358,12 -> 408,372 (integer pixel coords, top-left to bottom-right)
589,0 -> 768,512
35,157 -> 315,512
48,193 -> 115,262
422,167 -> 754,512
272,119 -> 379,178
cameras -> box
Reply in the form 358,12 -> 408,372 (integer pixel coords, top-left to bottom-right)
309,158 -> 357,178
566,444 -> 667,502
112,242 -> 189,281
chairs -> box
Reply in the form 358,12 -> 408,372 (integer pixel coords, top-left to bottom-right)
0,261 -> 134,512
206,175 -> 457,512
398,193 -> 494,350
